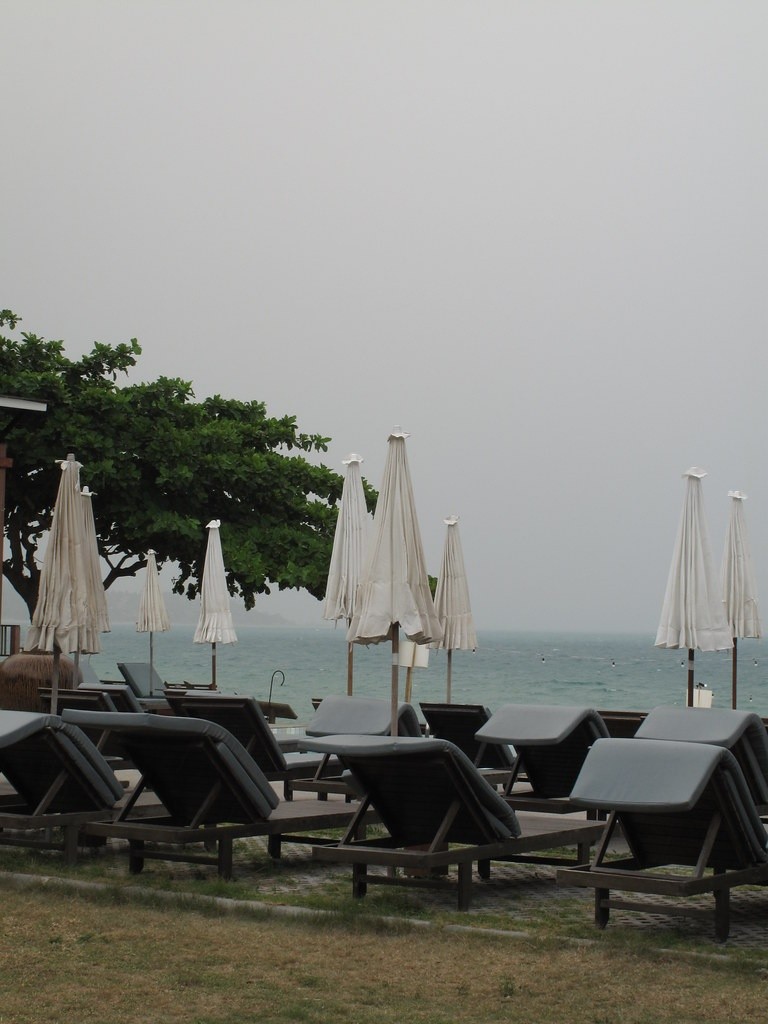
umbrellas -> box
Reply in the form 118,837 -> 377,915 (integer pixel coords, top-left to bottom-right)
193,520 -> 238,690
324,425 -> 478,739
136,550 -> 172,697
654,468 -> 762,709
26,454 -> 112,715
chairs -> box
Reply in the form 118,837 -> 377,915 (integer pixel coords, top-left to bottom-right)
0,662 -> 768,945
